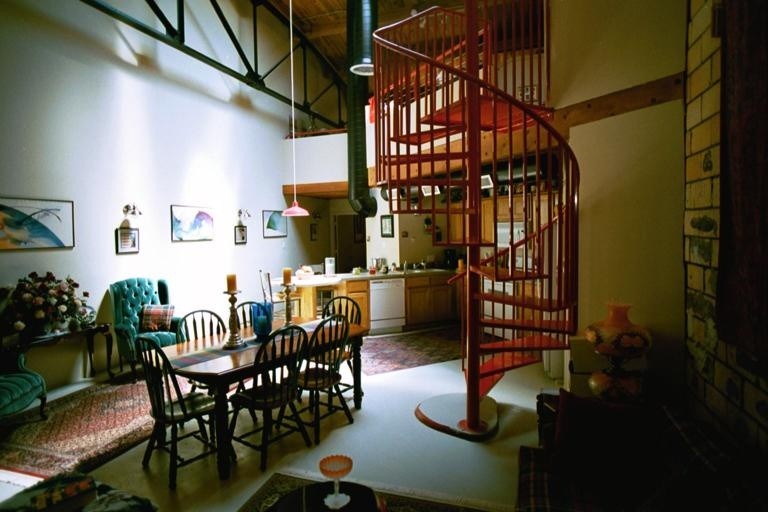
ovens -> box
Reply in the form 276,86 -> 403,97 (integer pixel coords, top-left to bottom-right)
482,278 -> 517,337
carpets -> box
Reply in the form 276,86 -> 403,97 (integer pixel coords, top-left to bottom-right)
1,374 -> 213,483
359,323 -> 511,377
236,473 -> 517,512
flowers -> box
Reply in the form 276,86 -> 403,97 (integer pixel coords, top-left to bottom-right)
0,271 -> 99,340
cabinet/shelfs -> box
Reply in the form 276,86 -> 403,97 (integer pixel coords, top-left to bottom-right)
339,279 -> 371,332
497,193 -> 533,222
403,272 -> 455,330
516,281 -> 542,338
447,197 -> 496,244
531,191 -> 561,338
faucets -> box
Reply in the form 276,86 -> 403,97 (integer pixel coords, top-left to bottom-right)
420,259 -> 427,269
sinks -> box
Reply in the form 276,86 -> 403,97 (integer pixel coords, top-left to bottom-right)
392,268 -> 452,275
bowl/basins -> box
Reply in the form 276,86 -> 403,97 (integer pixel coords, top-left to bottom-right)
296,272 -> 315,279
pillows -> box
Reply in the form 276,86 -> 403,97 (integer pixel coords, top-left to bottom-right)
545,385 -> 658,488
140,303 -> 174,332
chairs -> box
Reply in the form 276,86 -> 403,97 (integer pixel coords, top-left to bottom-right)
511,390 -> 767,510
226,323 -> 311,472
258,270 -> 294,313
108,277 -> 187,383
231,300 -> 302,424
274,313 -> 354,445
177,309 -> 259,444
133,334 -> 236,491
295,295 -> 365,413
0,349 -> 49,437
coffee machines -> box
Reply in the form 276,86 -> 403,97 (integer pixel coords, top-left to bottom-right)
444,248 -> 456,269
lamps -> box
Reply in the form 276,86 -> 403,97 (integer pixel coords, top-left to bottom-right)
237,208 -> 253,219
122,205 -> 143,216
280,1 -> 312,217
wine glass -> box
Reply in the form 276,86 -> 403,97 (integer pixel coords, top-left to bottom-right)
319,453 -> 353,509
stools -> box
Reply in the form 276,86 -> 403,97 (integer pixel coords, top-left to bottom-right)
0,467 -> 158,512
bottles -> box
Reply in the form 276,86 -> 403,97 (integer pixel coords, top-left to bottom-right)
421,260 -> 426,269
403,260 -> 408,271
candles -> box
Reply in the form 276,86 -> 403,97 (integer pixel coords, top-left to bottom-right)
226,274 -> 237,291
283,268 -> 291,284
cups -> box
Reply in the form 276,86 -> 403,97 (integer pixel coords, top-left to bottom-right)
381,265 -> 389,274
370,267 -> 376,274
352,267 -> 360,275
325,258 -> 335,277
252,303 -> 274,342
458,259 -> 464,272
391,263 -> 396,272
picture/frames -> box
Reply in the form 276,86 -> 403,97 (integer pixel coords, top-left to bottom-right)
115,227 -> 139,254
261,209 -> 288,238
234,227 -> 248,245
171,204 -> 215,241
310,223 -> 320,242
0,194 -> 76,251
380,213 -> 394,238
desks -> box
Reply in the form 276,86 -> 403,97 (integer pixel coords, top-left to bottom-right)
8,322 -> 117,420
261,478 -> 388,512
568,335 -> 659,403
